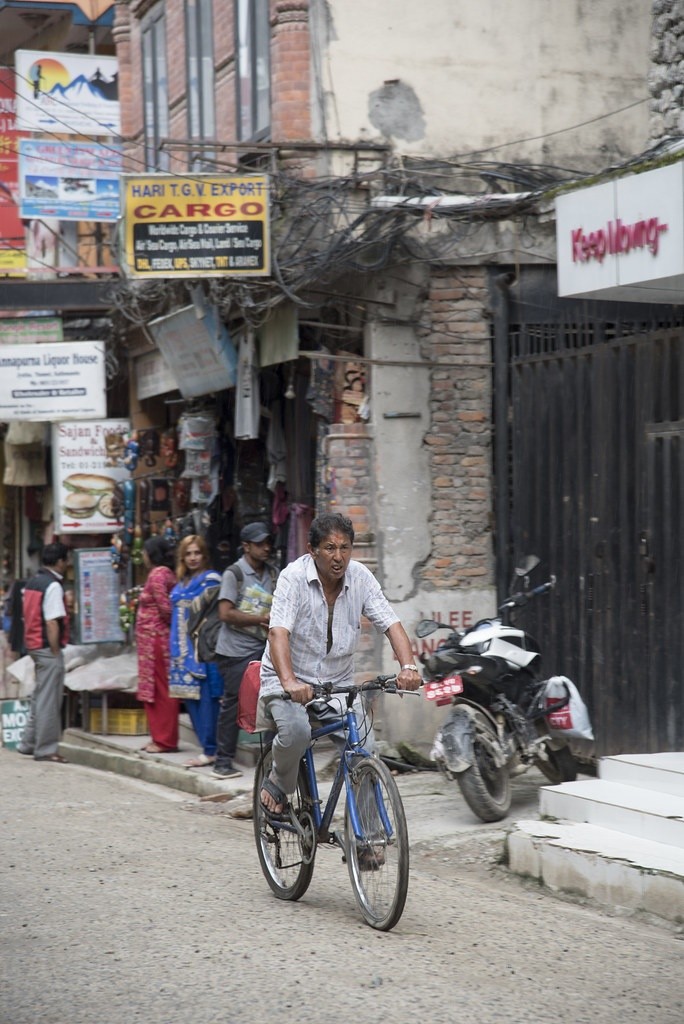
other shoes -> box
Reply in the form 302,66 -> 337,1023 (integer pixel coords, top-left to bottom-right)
210,766 -> 242,779
141,742 -> 179,753
182,752 -> 216,768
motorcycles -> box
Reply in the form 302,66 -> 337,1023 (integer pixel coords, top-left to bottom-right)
410,553 -> 583,821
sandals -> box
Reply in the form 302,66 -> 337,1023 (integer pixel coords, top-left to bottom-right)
35,754 -> 69,763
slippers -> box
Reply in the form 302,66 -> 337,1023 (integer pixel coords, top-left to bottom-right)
259,776 -> 288,819
342,850 -> 385,867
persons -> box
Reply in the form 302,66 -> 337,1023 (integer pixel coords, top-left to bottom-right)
212,521 -> 280,780
168,536 -> 223,768
18,543 -> 69,763
135,535 -> 180,754
259,514 -> 423,866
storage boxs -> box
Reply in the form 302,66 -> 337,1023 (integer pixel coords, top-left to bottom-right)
89,708 -> 150,735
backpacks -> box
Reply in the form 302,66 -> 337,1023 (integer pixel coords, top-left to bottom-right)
187,561 -> 280,662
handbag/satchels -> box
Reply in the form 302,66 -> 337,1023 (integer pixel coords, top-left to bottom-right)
541,676 -> 594,741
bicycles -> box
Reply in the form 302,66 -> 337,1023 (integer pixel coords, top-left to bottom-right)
249,675 -> 420,934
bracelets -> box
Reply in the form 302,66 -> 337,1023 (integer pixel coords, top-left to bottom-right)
402,664 -> 418,672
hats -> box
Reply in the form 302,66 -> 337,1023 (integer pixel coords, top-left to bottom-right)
240,521 -> 273,543
